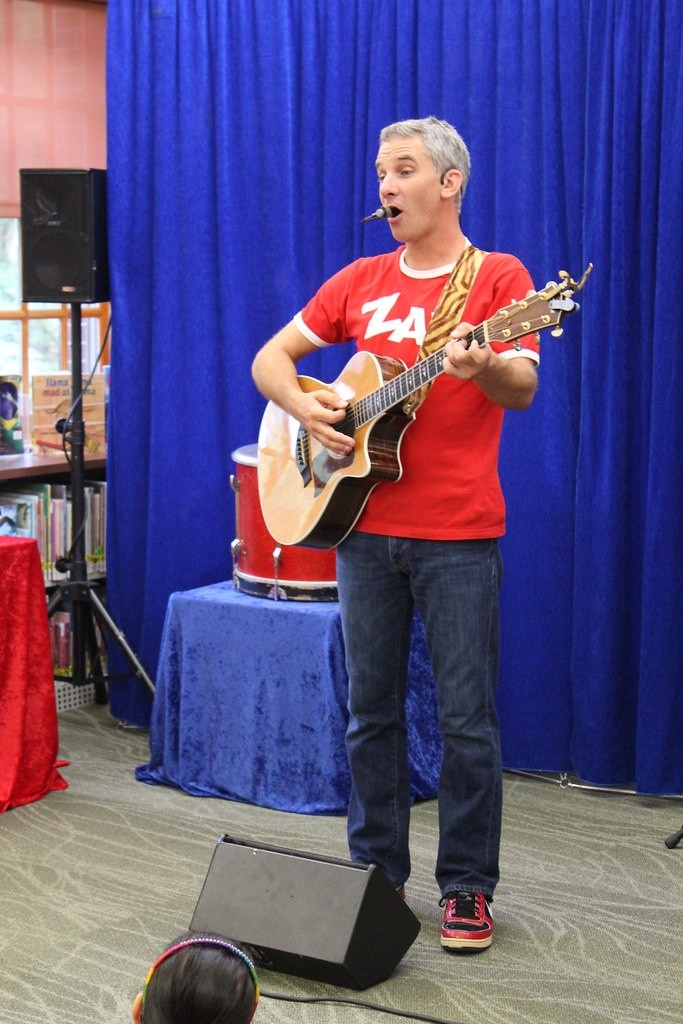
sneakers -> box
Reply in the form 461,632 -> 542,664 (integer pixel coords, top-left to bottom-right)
439,891 -> 494,951
395,883 -> 405,900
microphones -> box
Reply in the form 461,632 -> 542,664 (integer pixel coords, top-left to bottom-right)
361,207 -> 391,223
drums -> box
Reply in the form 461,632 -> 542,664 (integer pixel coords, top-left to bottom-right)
230,444 -> 342,603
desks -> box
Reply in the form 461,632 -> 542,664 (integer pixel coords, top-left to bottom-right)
134,578 -> 442,816
0,535 -> 70,813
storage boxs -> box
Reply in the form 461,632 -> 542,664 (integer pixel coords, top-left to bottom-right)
32,403 -> 105,435
31,372 -> 104,407
32,425 -> 107,456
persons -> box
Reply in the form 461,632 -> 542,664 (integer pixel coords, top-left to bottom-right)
251,116 -> 539,952
131,932 -> 259,1024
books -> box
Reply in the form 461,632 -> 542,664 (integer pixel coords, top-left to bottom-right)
0,477 -> 108,588
41,606 -> 112,684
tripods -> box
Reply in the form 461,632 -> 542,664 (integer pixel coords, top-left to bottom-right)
45,304 -> 157,705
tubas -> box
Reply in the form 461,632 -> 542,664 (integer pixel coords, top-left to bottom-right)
257,261 -> 594,551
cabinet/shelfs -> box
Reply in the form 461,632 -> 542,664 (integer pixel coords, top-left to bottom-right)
0,453 -> 107,655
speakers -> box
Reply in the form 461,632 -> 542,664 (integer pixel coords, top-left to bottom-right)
188,834 -> 421,991
19,168 -> 110,303
